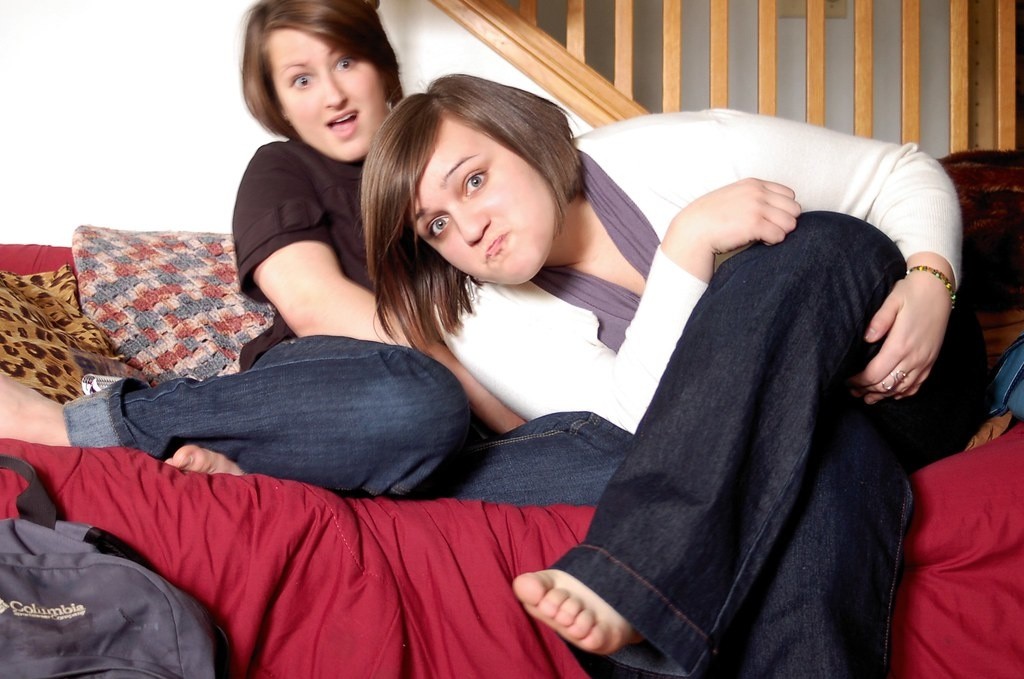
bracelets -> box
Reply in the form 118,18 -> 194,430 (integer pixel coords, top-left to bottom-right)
904,265 -> 956,310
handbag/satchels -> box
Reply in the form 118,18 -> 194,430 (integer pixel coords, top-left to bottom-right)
0,452 -> 231,679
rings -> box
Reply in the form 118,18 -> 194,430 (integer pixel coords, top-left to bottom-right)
882,370 -> 907,392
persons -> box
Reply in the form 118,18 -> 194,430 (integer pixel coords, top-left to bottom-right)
359,73 -> 994,679
0,0 -> 635,508
